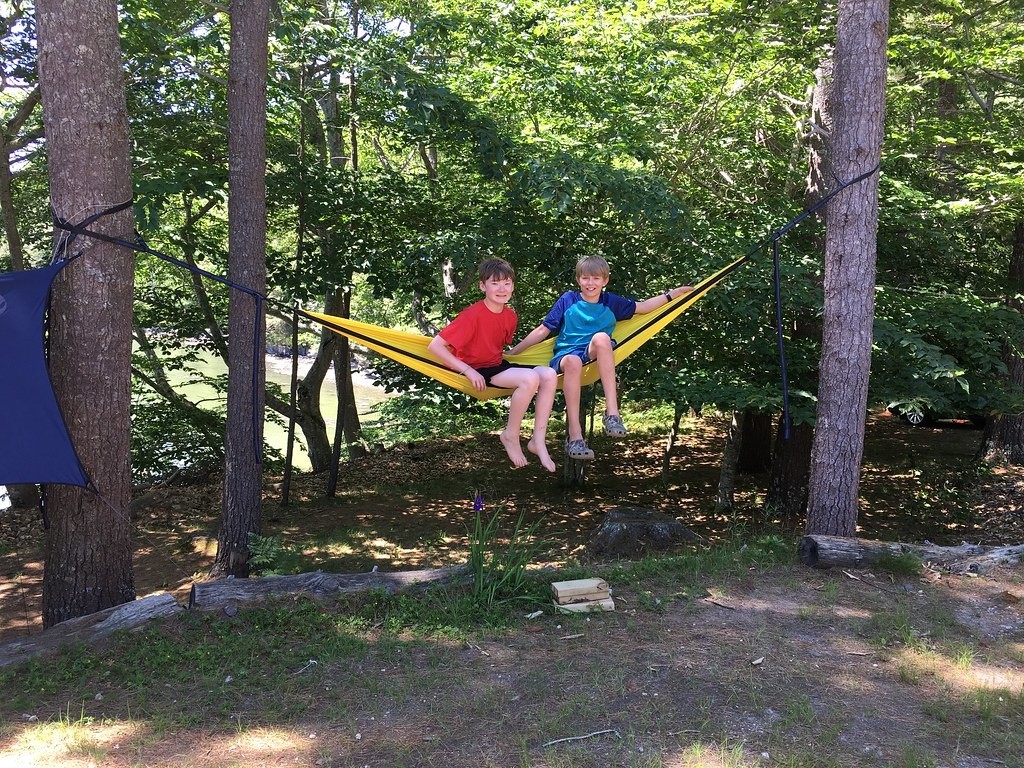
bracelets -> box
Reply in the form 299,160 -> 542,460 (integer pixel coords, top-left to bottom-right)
460,366 -> 470,374
664,291 -> 672,302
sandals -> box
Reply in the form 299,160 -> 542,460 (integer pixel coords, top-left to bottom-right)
602,409 -> 626,437
564,436 -> 594,459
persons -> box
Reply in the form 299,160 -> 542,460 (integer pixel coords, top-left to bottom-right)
428,257 -> 556,473
504,254 -> 695,458
